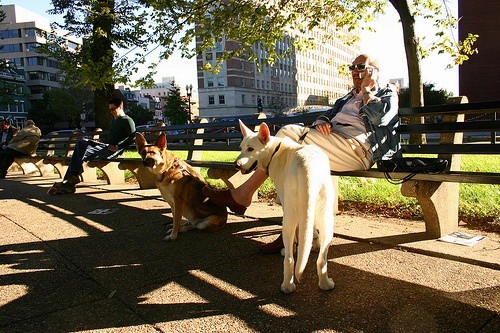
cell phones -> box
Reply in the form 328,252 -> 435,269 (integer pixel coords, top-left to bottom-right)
359,71 -> 367,79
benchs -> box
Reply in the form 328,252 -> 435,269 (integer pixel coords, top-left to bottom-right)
5,96 -> 500,237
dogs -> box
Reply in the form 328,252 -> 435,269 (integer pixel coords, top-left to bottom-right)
238,119 -> 335,294
134,131 -> 228,240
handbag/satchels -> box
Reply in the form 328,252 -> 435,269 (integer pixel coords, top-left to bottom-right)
383,143 -> 452,184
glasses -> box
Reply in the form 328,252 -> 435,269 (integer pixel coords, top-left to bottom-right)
349,65 -> 376,71
108,107 -> 117,112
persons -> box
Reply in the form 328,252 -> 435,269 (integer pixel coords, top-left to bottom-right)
202,54 -> 398,215
0,119 -> 41,179
58,98 -> 136,186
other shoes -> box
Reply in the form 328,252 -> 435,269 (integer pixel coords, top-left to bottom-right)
49,182 -> 76,194
68,174 -> 84,184
258,234 -> 285,255
203,185 -> 246,215
